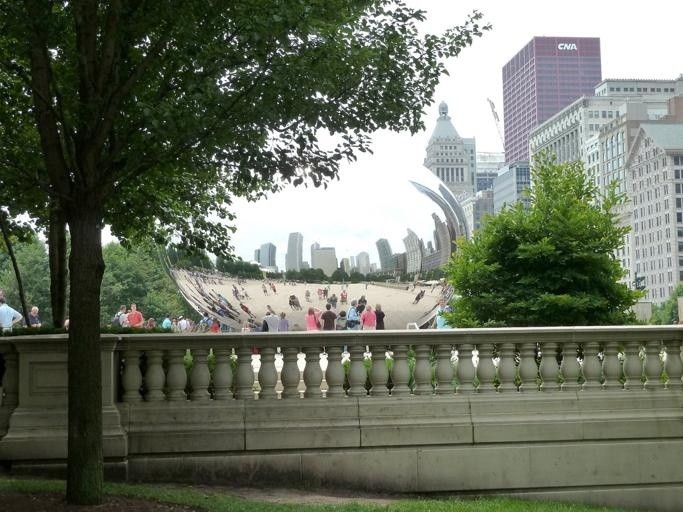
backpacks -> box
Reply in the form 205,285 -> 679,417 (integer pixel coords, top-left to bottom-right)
110,313 -> 124,327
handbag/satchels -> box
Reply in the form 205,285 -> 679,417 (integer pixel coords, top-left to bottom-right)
347,320 -> 354,328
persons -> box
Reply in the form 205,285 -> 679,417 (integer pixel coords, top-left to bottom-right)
259,300 -> 385,330
185,270 -> 452,322
1,297 -> 220,332
432,299 -> 452,330
